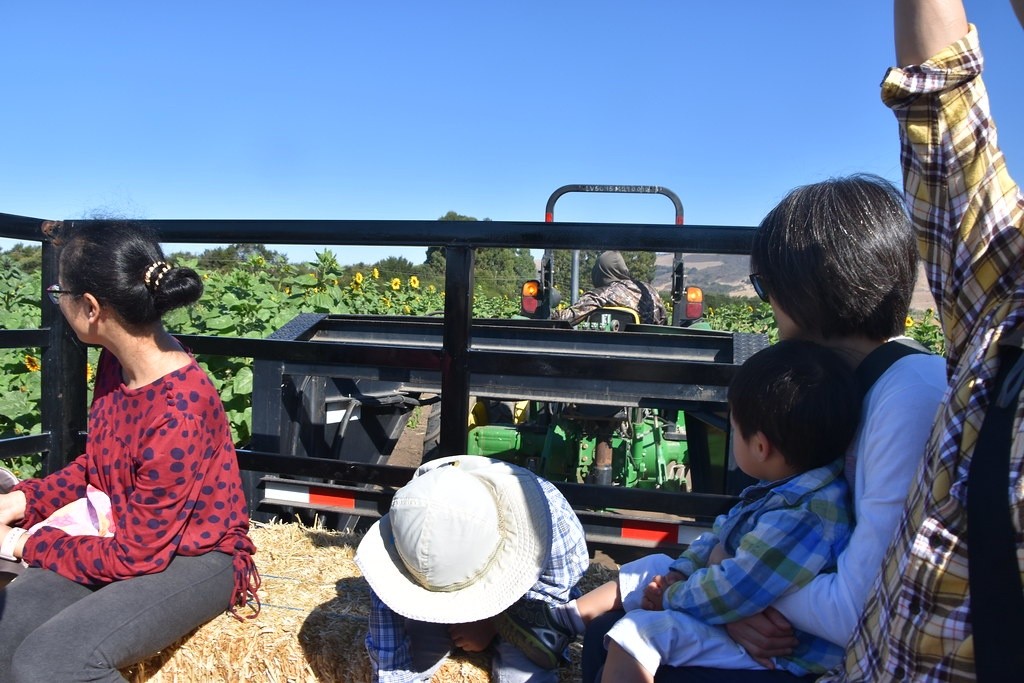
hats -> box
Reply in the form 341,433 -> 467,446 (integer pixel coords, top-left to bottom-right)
352,455 -> 550,623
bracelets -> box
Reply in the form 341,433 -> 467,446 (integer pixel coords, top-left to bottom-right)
0,527 -> 27,562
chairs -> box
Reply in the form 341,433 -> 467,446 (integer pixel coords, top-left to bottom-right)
588,306 -> 642,332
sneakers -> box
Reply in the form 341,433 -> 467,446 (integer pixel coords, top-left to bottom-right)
493,598 -> 577,670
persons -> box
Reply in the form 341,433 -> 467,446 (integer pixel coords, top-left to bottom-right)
2,225 -> 255,683
711,171 -> 950,674
353,451 -> 591,682
518,338 -> 855,683
833,0 -> 1024,683
556,250 -> 673,329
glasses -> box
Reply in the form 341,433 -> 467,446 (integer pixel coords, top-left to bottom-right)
48,284 -> 75,305
747,273 -> 769,304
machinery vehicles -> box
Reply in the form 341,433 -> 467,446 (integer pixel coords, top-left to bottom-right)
0,181 -> 776,683
